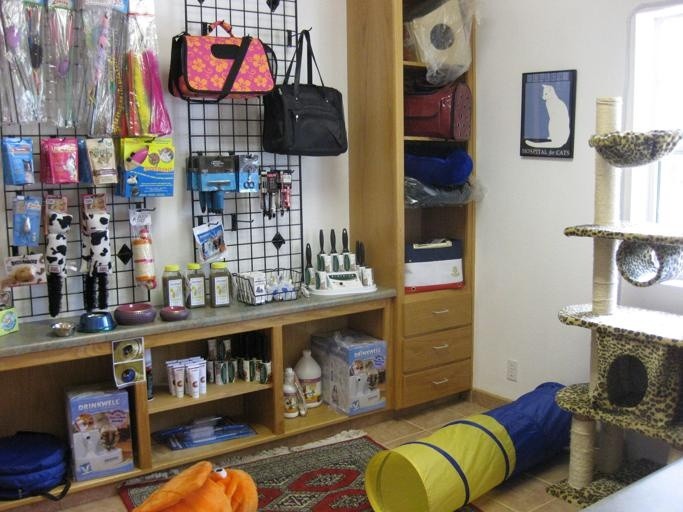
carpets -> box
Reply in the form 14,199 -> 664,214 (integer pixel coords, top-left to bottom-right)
114,426 -> 482,512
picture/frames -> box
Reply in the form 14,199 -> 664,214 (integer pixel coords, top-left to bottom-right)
519,69 -> 576,158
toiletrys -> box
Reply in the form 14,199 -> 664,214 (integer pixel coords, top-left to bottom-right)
208,358 -> 271,385
166,355 -> 207,399
166,356 -> 207,400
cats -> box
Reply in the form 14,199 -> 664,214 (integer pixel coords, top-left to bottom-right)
525,85 -> 570,148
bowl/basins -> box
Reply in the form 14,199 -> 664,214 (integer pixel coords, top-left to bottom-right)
160,305 -> 189,322
113,303 -> 157,325
75,311 -> 117,333
50,322 -> 76,337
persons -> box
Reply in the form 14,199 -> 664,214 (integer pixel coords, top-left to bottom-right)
350,360 -> 370,397
72,412 -> 106,460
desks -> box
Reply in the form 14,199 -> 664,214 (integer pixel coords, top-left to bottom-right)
580,455 -> 683,512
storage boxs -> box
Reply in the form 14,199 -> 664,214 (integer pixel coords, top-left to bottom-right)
403,258 -> 463,294
311,327 -> 387,416
64,381 -> 134,483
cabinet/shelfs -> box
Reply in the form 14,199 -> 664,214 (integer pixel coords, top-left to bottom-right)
0,300 -> 391,512
347,0 -> 476,418
548,93 -> 683,504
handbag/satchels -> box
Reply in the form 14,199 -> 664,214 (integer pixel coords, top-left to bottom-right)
261,84 -> 348,156
404,78 -> 473,141
0,431 -> 71,500
169,20 -> 276,100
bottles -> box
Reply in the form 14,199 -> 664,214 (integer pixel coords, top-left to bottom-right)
162,261 -> 205,309
209,262 -> 230,307
282,368 -> 300,418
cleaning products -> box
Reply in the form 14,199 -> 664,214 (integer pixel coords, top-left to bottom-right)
284,367 -> 300,419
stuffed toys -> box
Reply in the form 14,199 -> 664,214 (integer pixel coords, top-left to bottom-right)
131,456 -> 258,512
